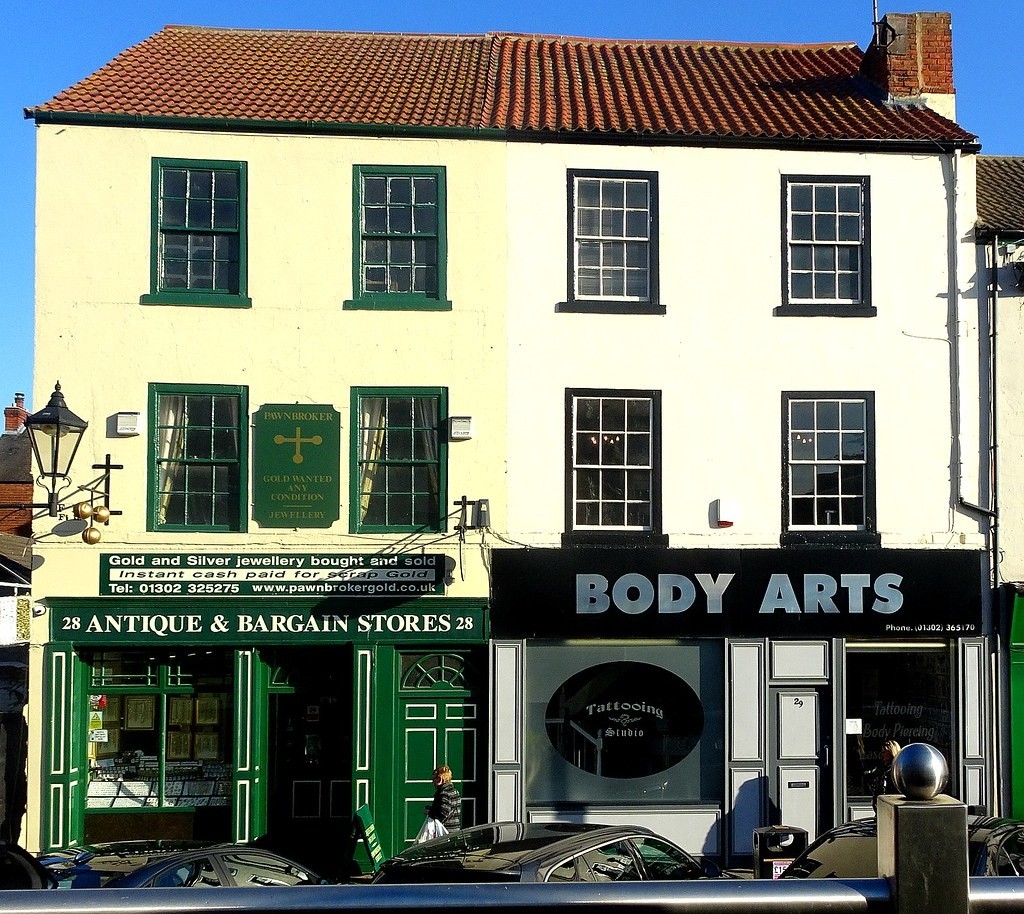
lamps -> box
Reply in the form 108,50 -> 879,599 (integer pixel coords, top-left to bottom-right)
19,379 -> 91,517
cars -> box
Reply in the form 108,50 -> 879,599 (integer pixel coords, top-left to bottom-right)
371,822 -> 721,883
778,814 -> 1024,877
34,839 -> 333,890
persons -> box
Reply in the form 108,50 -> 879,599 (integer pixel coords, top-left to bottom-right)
871,740 -> 901,817
847,734 -> 870,796
424,766 -> 461,834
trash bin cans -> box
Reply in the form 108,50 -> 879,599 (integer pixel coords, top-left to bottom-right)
752,826 -> 808,879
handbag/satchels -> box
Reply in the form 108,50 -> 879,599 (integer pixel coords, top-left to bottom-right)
412,815 -> 449,847
